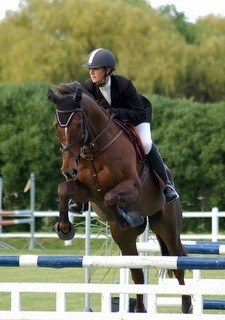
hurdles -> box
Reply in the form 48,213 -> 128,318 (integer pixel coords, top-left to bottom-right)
1,240 -> 225,320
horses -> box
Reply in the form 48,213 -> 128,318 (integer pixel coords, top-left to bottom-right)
48,81 -> 193,313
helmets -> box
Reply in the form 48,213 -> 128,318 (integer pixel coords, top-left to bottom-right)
82,48 -> 116,68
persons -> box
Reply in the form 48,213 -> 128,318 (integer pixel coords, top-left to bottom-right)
69,48 -> 180,215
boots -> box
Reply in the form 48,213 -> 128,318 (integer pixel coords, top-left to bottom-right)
146,144 -> 179,205
68,201 -> 88,212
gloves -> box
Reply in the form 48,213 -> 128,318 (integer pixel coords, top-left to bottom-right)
109,108 -> 120,119
